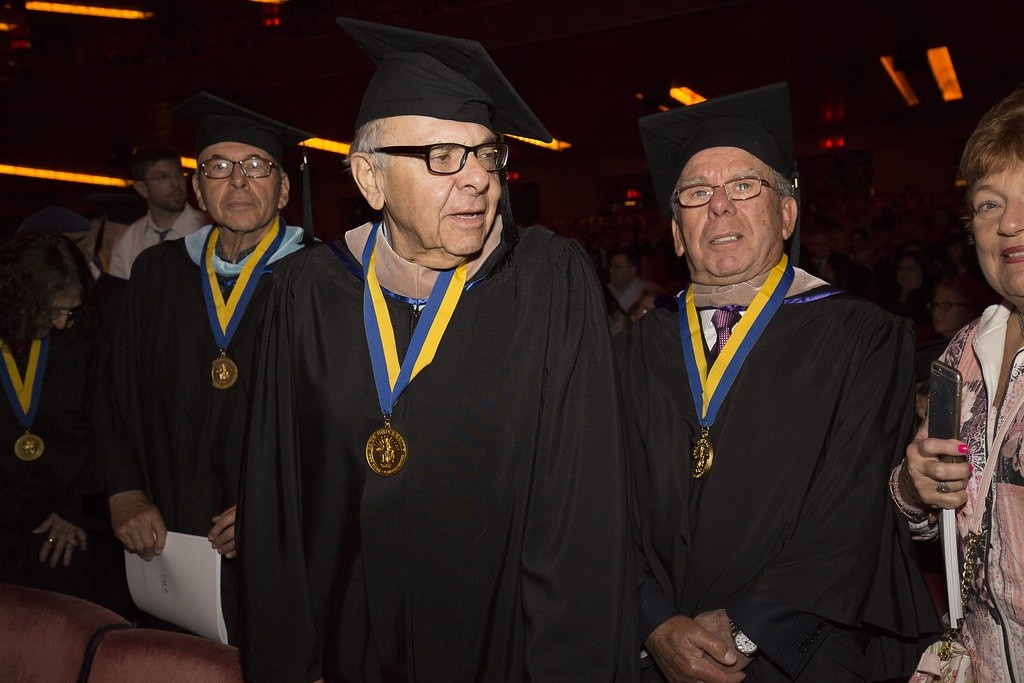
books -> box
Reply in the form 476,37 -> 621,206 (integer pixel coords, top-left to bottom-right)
124,530 -> 228,645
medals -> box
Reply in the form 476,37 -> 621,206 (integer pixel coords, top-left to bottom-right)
365,428 -> 407,476
693,439 -> 713,478
211,358 -> 238,390
14,434 -> 44,460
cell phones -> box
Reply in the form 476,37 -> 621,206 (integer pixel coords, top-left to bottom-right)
926,359 -> 963,466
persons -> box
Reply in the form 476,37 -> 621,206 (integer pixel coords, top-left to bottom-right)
819,228 -> 984,339
234,17 -> 641,683
889,87 -> 1024,683
0,206 -> 128,620
108,145 -> 207,281
619,82 -> 917,683
80,92 -> 322,645
589,227 -> 687,336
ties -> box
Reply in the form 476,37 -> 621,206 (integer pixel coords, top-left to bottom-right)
709,305 -> 742,366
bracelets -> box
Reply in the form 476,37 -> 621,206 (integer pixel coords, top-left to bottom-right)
888,464 -> 929,523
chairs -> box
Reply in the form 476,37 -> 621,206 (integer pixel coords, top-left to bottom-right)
0,584 -> 246,683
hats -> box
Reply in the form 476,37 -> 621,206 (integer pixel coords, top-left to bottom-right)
16,206 -> 92,234
168,92 -> 316,247
336,17 -> 551,288
638,81 -> 801,270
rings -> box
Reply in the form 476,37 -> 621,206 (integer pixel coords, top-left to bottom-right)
69,540 -> 79,546
47,538 -> 56,544
936,481 -> 950,493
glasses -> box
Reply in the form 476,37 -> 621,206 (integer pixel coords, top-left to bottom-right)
926,301 -> 964,312
39,308 -> 75,322
198,158 -> 279,179
143,169 -> 187,183
366,143 -> 510,173
672,176 -> 777,208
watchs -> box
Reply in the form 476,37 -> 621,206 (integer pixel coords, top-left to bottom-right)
729,618 -> 757,657
